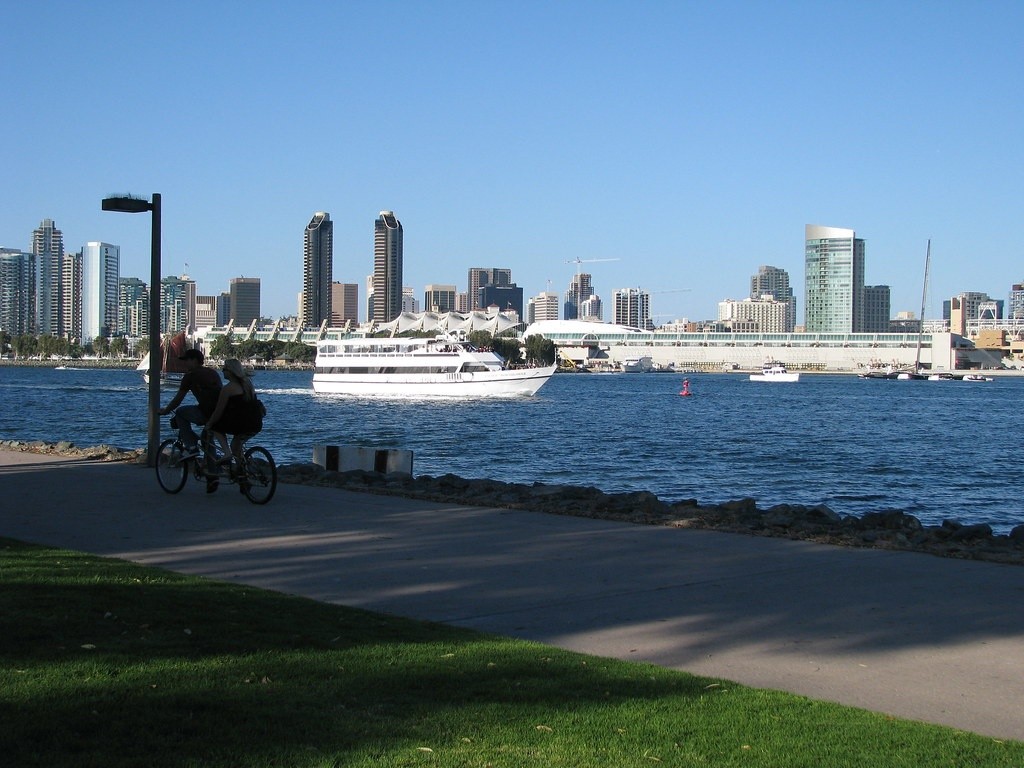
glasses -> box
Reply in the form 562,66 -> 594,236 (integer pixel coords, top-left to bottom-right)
222,368 -> 227,372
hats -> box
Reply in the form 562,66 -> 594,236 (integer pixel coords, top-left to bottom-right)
178,349 -> 204,361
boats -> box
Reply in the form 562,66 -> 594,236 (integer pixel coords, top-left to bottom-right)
312,328 -> 558,400
558,353 -> 627,373
749,364 -> 800,382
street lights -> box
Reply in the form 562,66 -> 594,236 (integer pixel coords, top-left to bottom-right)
101,192 -> 163,466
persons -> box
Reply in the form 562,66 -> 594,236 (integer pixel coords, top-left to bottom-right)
200,358 -> 267,496
156,349 -> 223,494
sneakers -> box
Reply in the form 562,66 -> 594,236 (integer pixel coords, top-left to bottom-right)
207,476 -> 220,494
177,446 -> 200,462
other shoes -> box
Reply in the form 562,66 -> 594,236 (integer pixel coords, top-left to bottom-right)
240,481 -> 252,494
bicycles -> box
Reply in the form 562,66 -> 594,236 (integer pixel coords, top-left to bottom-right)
155,406 -> 277,506
